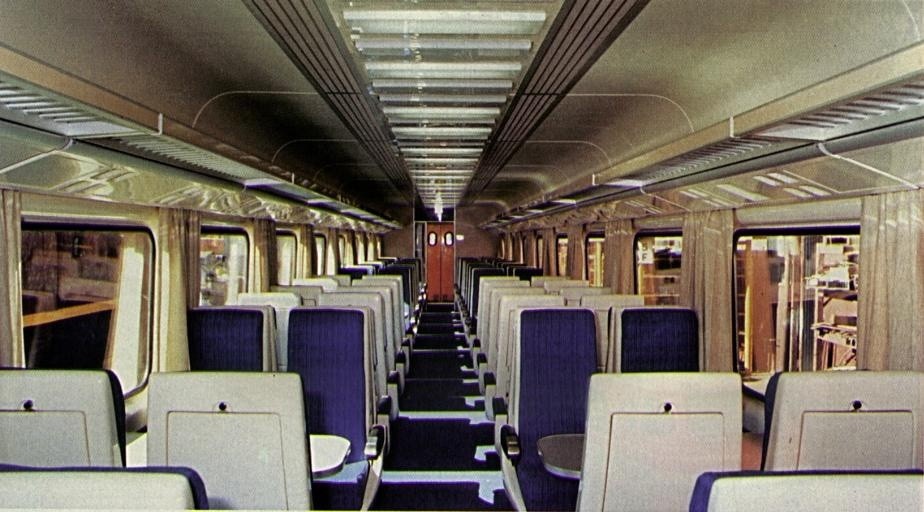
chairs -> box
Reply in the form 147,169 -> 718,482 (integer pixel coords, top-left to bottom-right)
146,372 -> 314,509
183,292 -> 399,509
453,257 -> 543,336
471,274 -> 646,422
574,373 -> 743,510
493,306 -> 700,511
1,368 -> 128,510
2,464 -> 210,510
689,469 -> 924,511
758,371 -> 923,471
269,255 -> 424,394
